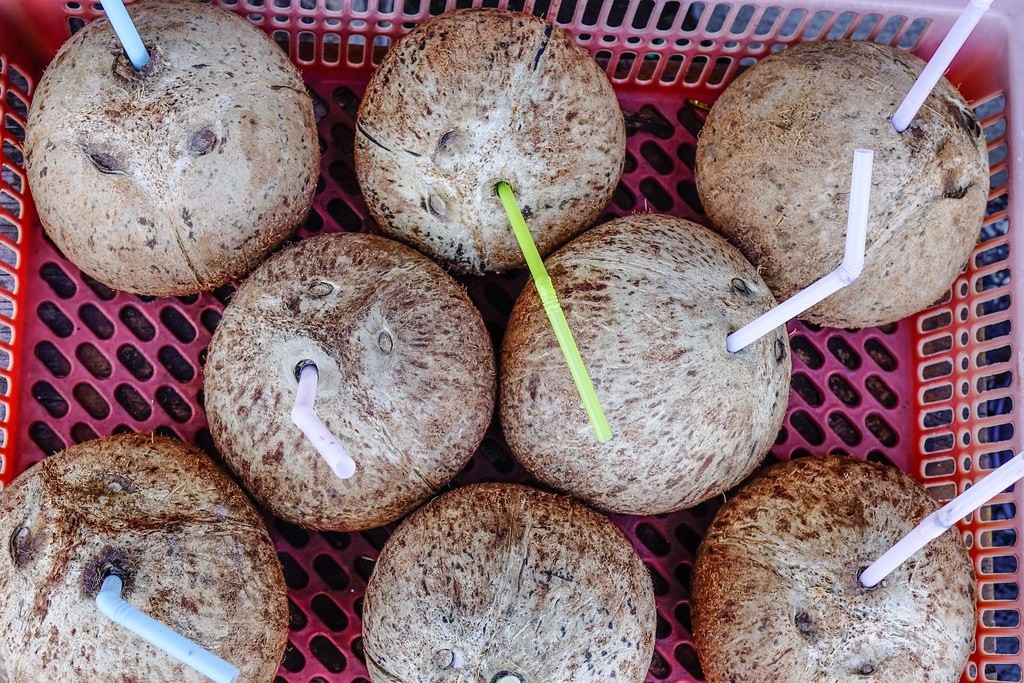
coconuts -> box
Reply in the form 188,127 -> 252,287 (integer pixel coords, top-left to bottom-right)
362,484 -> 657,683
24,0 -> 321,298
497,213 -> 792,516
202,232 -> 497,532
354,8 -> 625,277
694,38 -> 990,329
0,432 -> 289,683
691,455 -> 977,683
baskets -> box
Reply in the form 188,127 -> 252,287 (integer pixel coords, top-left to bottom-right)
0,0 -> 1024,683
